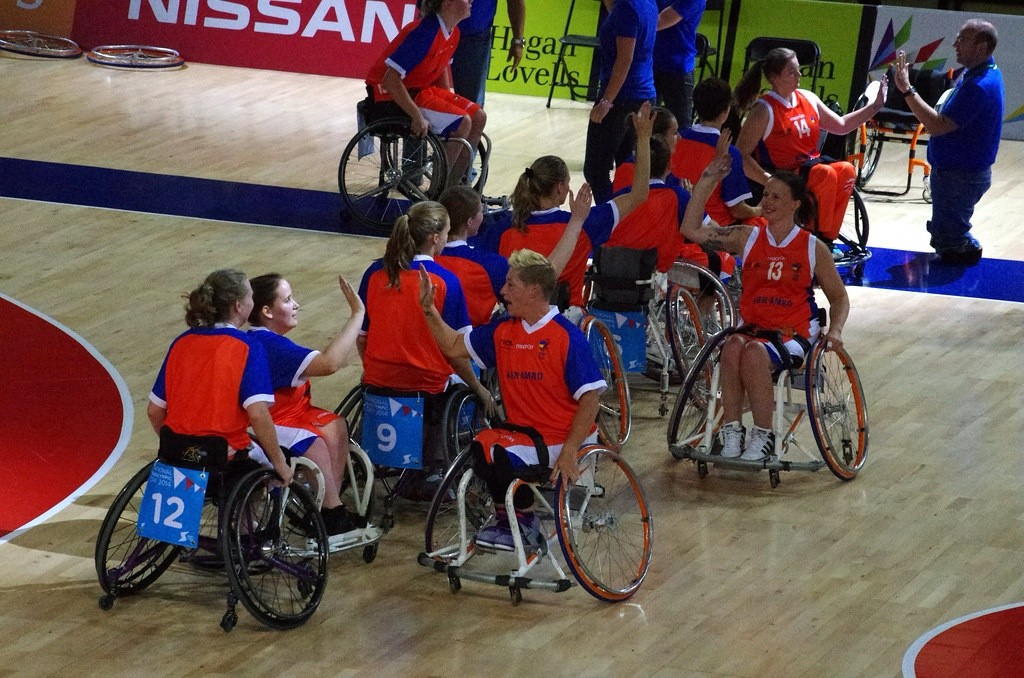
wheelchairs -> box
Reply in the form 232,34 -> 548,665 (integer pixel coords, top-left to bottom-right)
331,374 -> 495,532
474,150 -> 873,453
95,422 -> 332,633
662,311 -> 875,482
415,412 -> 656,607
337,80 -> 494,232
243,421 -> 386,567
850,64 -> 958,204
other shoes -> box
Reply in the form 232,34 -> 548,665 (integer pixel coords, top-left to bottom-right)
320,501 -> 367,533
398,180 -> 421,202
426,460 -> 447,497
685,316 -> 722,338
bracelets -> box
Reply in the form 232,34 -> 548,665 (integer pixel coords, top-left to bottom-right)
600,98 -> 612,108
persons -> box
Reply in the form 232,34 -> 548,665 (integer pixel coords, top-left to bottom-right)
584,0 -> 659,204
403,0 -> 526,183
246,272 -> 370,535
608,49 -> 889,369
418,249 -> 607,549
680,152 -> 850,460
147,271 -> 293,548
652,0 -> 707,132
365,0 -> 486,198
895,20 -> 1004,263
356,100 -> 657,475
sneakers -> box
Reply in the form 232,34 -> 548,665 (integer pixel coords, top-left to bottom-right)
721,421 -> 747,458
475,511 -> 508,549
495,509 -> 540,550
741,426 -> 774,463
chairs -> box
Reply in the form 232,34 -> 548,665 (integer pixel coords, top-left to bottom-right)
695,0 -> 726,90
546,0 -> 607,109
740,35 -> 821,93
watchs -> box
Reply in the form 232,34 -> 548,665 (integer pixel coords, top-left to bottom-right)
511,37 -> 526,48
903,86 -> 916,98
